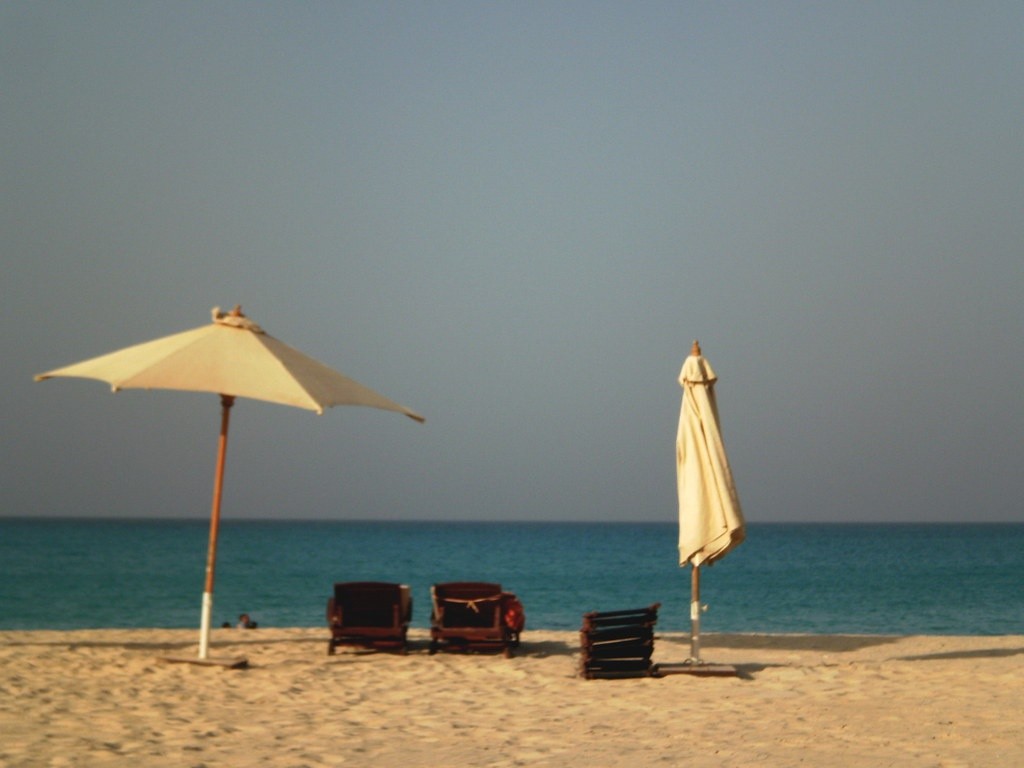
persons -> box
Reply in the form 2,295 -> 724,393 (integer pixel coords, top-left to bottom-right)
217,611 -> 262,630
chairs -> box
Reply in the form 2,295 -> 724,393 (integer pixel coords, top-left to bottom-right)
579,602 -> 660,680
327,581 -> 526,658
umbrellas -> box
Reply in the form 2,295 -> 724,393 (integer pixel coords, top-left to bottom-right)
30,301 -> 428,664
670,338 -> 749,665
